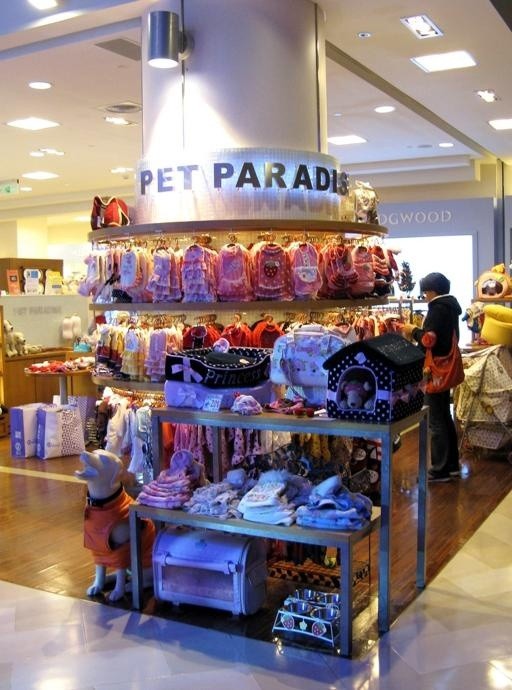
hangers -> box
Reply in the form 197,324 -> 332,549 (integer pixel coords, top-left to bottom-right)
93,305 -> 411,331
88,235 -> 392,256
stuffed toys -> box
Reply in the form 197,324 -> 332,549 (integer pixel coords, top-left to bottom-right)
2,320 -> 20,357
336,379 -> 376,411
12,332 -> 30,356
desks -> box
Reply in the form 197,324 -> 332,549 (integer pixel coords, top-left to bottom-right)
129,505 -> 383,660
25,370 -> 92,404
150,405 -> 431,633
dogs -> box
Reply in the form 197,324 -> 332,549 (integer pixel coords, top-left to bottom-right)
73,447 -> 159,603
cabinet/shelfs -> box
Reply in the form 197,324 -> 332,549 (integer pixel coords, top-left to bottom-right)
0,354 -> 66,407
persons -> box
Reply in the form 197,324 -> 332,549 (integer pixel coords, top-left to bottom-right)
398,271 -> 462,484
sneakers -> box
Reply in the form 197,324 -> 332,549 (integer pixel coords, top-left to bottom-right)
417,467 -> 460,483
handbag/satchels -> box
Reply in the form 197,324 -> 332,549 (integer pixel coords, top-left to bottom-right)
10,402 -> 85,460
419,345 -> 465,395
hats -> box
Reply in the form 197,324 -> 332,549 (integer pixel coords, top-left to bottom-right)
420,272 -> 450,293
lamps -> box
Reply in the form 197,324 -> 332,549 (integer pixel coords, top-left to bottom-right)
147,11 -> 194,69
